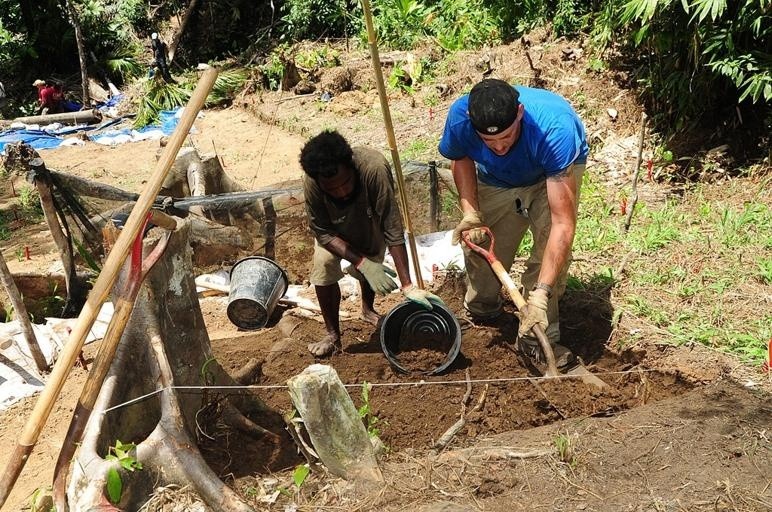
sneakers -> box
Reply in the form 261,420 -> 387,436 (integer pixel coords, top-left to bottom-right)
455,308 -> 504,330
514,336 -> 574,367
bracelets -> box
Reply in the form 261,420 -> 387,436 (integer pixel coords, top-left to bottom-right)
528,280 -> 555,299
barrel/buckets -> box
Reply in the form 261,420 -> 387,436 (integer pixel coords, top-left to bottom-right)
379,301 -> 463,378
227,257 -> 289,330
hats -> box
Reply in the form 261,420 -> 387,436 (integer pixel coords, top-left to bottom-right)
151,32 -> 158,40
468,78 -> 519,135
32,79 -> 46,87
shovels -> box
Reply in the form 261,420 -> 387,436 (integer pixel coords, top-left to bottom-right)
462,226 -> 625,420
34,210 -> 177,512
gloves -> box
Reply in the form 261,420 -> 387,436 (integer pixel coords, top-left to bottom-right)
518,290 -> 549,338
451,210 -> 490,247
357,257 -> 445,311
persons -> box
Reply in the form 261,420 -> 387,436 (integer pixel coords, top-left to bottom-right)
436,78 -> 588,372
298,125 -> 446,357
31,79 -> 58,115
